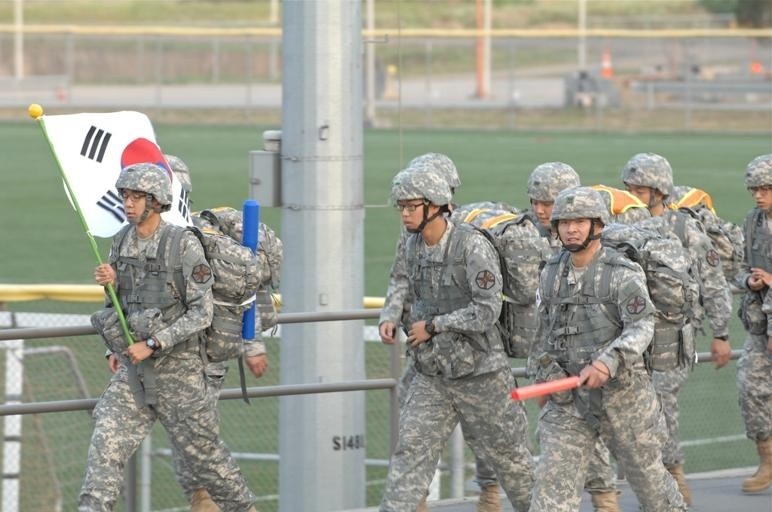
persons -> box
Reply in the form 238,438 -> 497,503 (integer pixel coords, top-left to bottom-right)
378,162 -> 539,512
729,152 -> 772,492
100,151 -> 271,512
620,151 -> 732,512
524,185 -> 691,512
73,160 -> 264,512
405,153 -> 505,512
524,161 -> 621,512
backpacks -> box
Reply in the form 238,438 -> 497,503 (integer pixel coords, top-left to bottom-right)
200,206 -> 285,331
591,185 -> 650,225
603,216 -> 702,372
458,208 -> 545,358
203,228 -> 265,360
666,186 -> 745,281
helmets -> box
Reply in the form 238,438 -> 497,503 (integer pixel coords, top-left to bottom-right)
409,153 -> 462,188
623,152 -> 674,197
116,162 -> 173,208
551,187 -> 610,226
526,161 -> 581,201
392,167 -> 454,208
745,154 -> 772,188
164,154 -> 191,196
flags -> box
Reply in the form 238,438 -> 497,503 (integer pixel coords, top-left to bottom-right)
43,109 -> 194,240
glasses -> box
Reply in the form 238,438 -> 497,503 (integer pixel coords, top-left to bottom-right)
395,201 -> 427,212
121,192 -> 146,202
748,187 -> 772,196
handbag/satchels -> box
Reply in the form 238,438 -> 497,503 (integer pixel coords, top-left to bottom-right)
648,319 -> 698,371
406,330 -> 475,381
534,353 -> 575,405
91,307 -> 175,357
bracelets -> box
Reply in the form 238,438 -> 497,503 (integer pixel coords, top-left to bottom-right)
713,334 -> 729,341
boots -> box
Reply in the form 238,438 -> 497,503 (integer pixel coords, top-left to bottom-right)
667,463 -> 693,508
189,489 -> 223,512
590,489 -> 620,512
741,438 -> 772,493
417,494 -> 426,512
476,486 -> 501,512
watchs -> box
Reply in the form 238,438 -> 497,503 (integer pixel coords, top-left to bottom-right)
145,336 -> 158,351
424,319 -> 438,337
